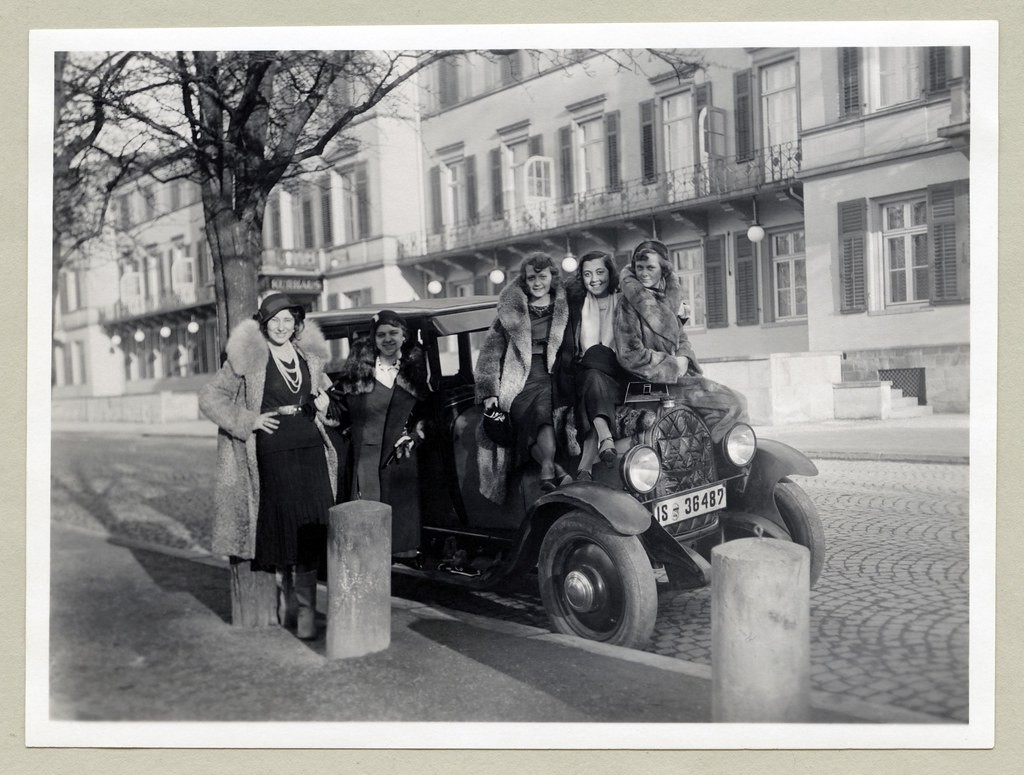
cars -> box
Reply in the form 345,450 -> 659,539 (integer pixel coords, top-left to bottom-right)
297,294 -> 828,652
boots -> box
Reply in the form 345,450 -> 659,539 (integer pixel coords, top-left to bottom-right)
276,566 -> 318,639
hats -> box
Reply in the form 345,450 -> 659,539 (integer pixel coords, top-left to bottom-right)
367,310 -> 409,331
631,239 -> 669,266
579,345 -> 619,380
256,293 -> 305,327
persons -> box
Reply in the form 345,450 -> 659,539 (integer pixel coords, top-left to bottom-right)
612,241 -> 751,444
554,250 -> 621,481
200,293 -> 332,640
474,252 -> 574,493
329,310 -> 432,565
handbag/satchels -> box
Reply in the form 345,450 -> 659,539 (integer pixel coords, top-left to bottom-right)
483,403 -> 514,450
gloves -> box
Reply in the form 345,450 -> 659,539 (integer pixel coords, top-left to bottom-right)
302,381 -> 348,421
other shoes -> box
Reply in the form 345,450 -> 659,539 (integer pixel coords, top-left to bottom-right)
598,438 -> 618,470
575,468 -> 593,481
540,473 -> 573,491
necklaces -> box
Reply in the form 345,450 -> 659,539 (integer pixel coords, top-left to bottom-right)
266,340 -> 302,394
376,351 -> 397,371
529,300 -> 553,317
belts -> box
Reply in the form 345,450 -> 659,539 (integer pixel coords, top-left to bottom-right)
260,404 -> 303,417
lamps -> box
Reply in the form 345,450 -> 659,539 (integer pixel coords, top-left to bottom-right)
111,331 -> 122,344
134,327 -> 145,342
489,252 -> 504,284
562,237 -> 578,273
160,321 -> 171,338
427,264 -> 441,295
747,195 -> 765,243
188,314 -> 199,333
